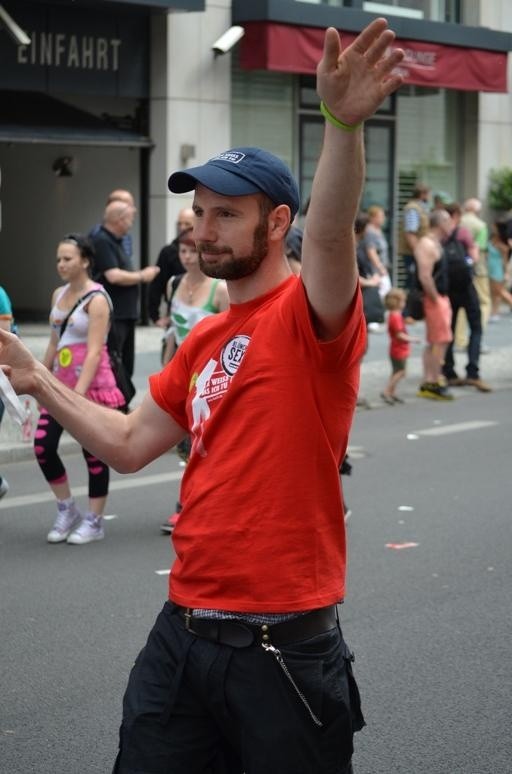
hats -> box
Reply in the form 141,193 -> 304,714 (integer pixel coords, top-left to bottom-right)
167,147 -> 300,224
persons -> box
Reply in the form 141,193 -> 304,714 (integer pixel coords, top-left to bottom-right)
353,185 -> 512,409
81,191 -> 230,462
0,17 -> 405,773
0,286 -> 19,500
33,234 -> 125,544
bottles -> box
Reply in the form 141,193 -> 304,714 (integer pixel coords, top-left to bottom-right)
21,401 -> 33,442
465,255 -> 474,279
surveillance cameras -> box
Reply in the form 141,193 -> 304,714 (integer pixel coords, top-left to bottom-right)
210,26 -> 246,55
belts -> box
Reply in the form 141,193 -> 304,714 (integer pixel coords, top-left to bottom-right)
174,604 -> 338,648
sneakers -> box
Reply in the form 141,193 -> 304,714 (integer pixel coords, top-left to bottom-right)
45,494 -> 82,544
379,392 -> 404,407
67,512 -> 106,545
416,375 -> 493,402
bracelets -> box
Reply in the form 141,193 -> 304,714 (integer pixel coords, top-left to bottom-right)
320,101 -> 365,132
